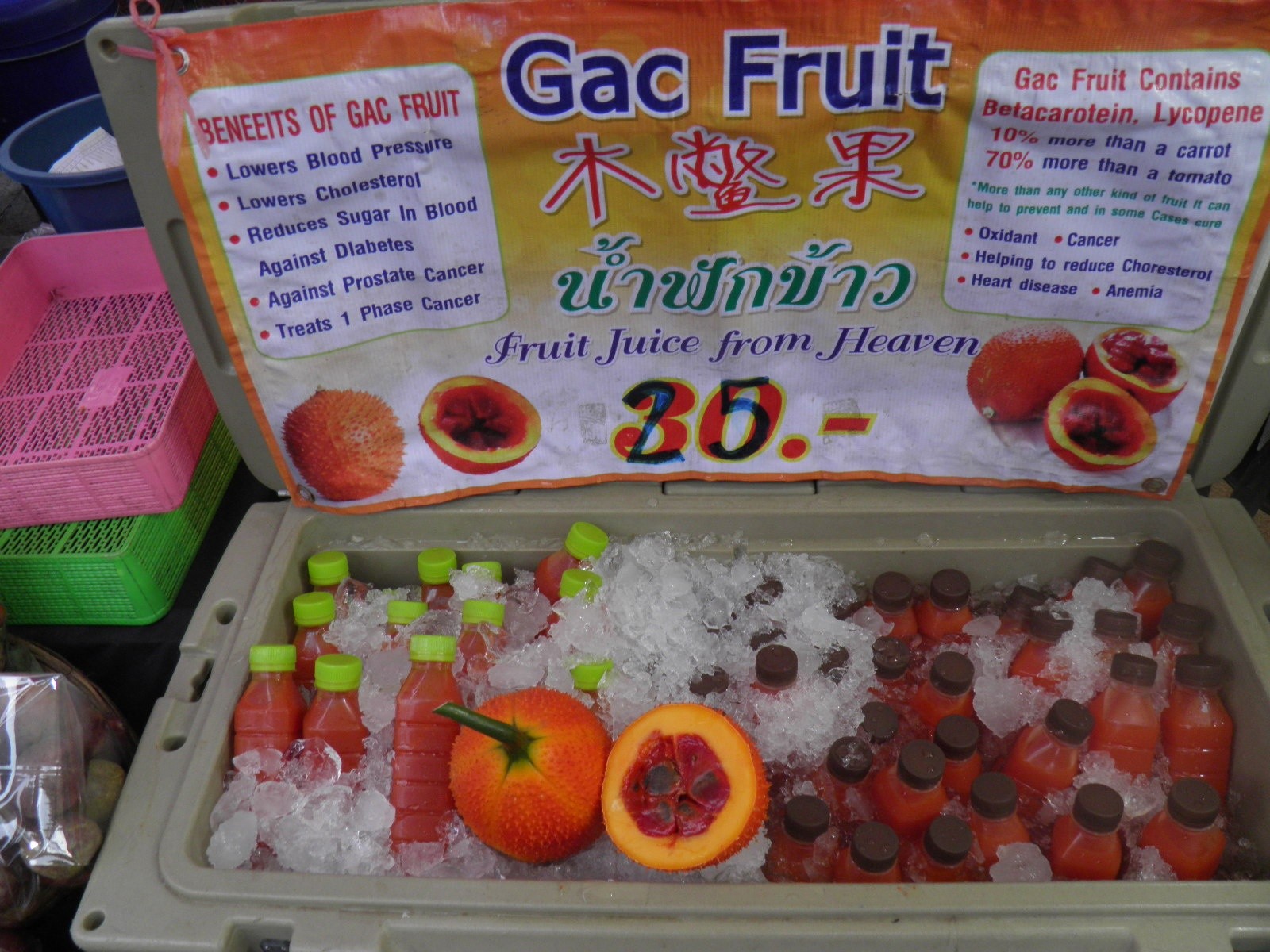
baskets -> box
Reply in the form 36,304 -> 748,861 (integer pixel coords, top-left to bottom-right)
1,228 -> 217,528
0,410 -> 245,627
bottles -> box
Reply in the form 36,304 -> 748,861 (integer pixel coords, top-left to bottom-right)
232,523 -> 617,870
676,543 -> 1238,884
